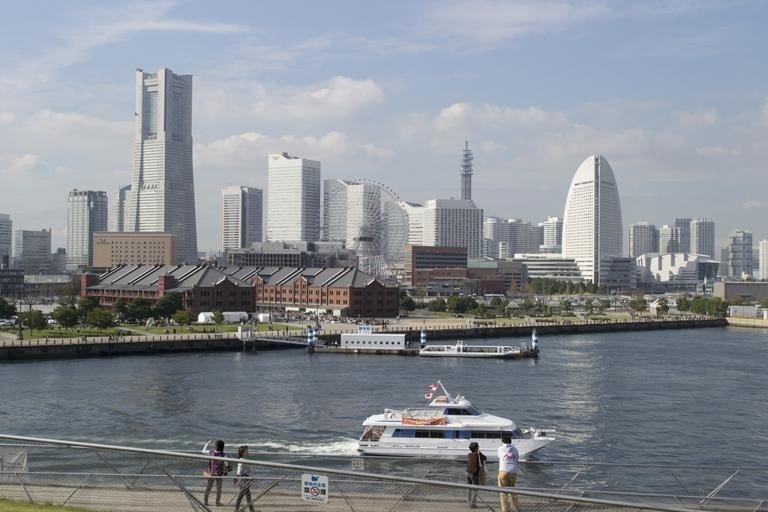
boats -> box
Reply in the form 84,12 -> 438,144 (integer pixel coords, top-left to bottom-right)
418,341 -> 538,358
356,380 -> 556,462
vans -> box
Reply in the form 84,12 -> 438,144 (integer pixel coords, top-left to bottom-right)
0,316 -> 23,326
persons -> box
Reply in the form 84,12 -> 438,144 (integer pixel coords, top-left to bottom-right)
235,446 -> 260,512
466,442 -> 487,509
202,439 -> 228,506
498,436 -> 519,512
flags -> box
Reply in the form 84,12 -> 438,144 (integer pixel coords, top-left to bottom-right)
425,393 -> 432,398
426,383 -> 438,391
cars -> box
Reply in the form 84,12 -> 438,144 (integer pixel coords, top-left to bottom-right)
42,315 -> 60,324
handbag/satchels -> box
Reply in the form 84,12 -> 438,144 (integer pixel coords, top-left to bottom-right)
223,462 -> 232,475
204,472 -> 212,480
480,464 -> 488,479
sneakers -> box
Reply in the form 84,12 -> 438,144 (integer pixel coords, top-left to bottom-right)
216,503 -> 223,506
